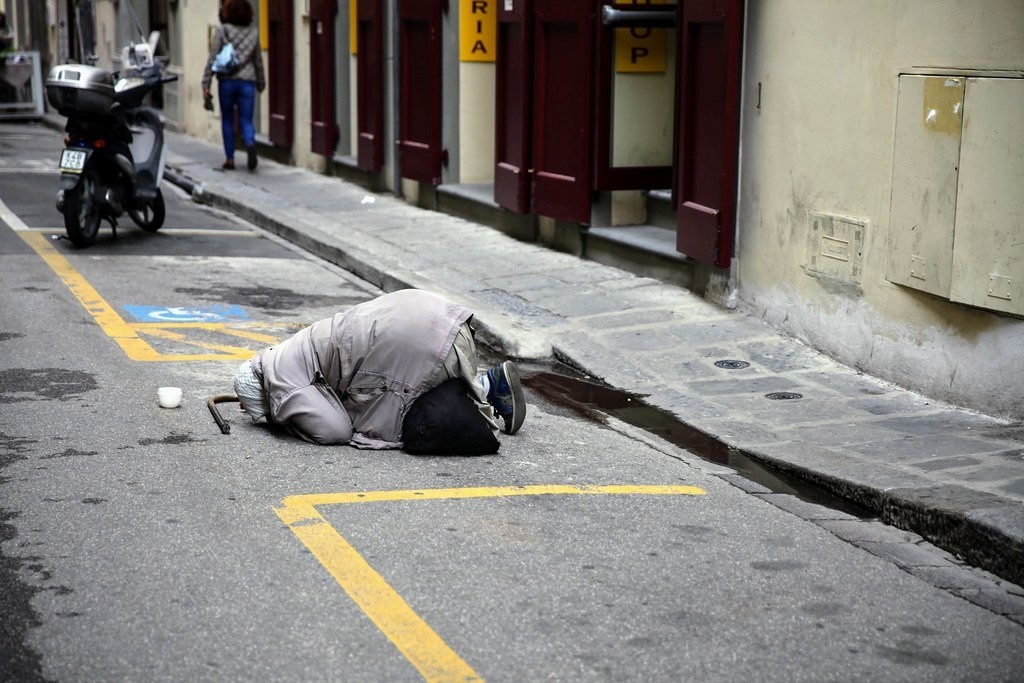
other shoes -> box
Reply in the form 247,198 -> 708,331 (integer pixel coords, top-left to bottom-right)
223,160 -> 235,170
248,147 -> 257,170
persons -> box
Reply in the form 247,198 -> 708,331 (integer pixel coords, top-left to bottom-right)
202,1 -> 266,172
234,289 -> 527,450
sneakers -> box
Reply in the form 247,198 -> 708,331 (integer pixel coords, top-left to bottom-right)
486,361 -> 527,433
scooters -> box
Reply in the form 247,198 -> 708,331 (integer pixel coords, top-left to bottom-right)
44,30 -> 178,250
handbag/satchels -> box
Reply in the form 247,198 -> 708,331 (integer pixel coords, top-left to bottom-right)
211,26 -> 241,75
